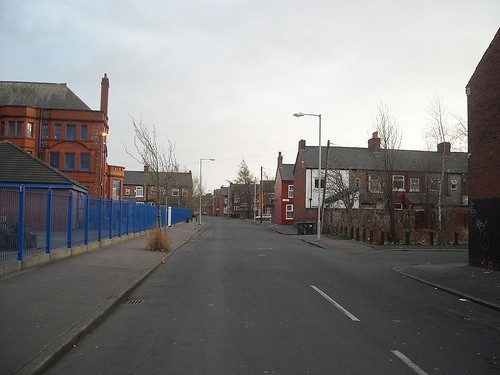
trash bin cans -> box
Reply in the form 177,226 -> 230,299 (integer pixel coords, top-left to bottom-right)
296,221 -> 305,234
305,221 -> 314,234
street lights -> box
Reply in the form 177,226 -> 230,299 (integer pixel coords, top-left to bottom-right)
199,158 -> 215,225
293,113 -> 323,242
